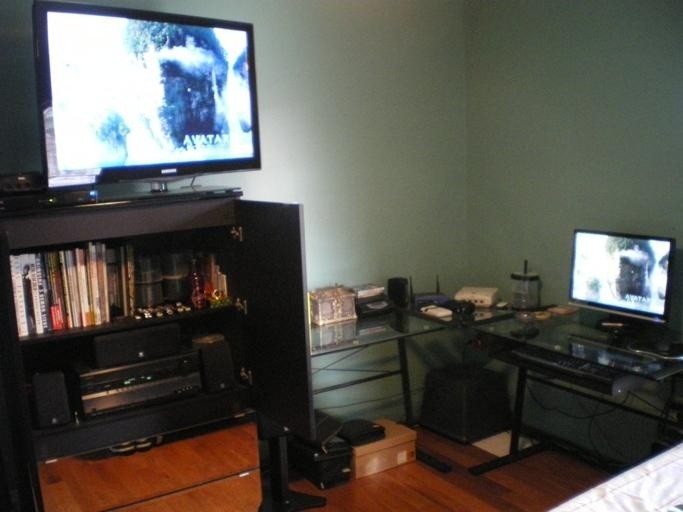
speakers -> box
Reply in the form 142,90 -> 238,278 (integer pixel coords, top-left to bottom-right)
86,322 -> 177,369
191,334 -> 235,394
31,369 -> 72,428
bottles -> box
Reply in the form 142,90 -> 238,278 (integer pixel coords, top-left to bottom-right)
186,258 -> 207,310
512,260 -> 540,308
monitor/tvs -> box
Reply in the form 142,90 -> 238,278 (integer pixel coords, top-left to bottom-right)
31,0 -> 262,206
568,228 -> 677,337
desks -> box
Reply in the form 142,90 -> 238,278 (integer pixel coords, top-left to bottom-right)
307,301 -> 683,485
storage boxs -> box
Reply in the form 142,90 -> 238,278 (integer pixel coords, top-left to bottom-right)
309,286 -> 358,326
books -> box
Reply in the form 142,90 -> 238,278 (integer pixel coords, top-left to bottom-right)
7,238 -> 229,340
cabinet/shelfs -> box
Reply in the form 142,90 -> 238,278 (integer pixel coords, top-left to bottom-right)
0,190 -> 264,512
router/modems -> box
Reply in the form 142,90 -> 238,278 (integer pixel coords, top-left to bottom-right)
408,275 -> 446,307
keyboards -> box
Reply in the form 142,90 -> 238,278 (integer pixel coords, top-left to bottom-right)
510,346 -> 626,384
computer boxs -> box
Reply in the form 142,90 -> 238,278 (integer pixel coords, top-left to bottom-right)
651,400 -> 683,452
